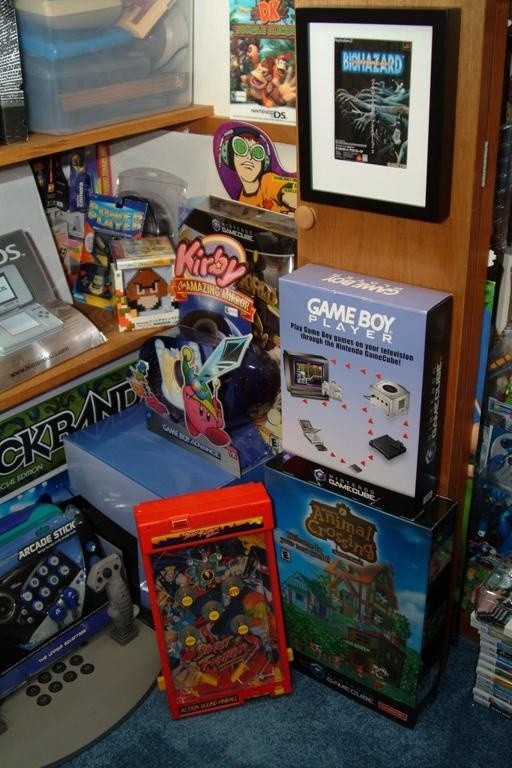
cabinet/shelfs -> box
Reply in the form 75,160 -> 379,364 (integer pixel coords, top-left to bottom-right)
1,104 -> 214,414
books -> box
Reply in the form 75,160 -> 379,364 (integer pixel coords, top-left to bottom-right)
471,610 -> 511,720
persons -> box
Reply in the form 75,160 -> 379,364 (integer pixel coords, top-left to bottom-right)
336,79 -> 409,169
